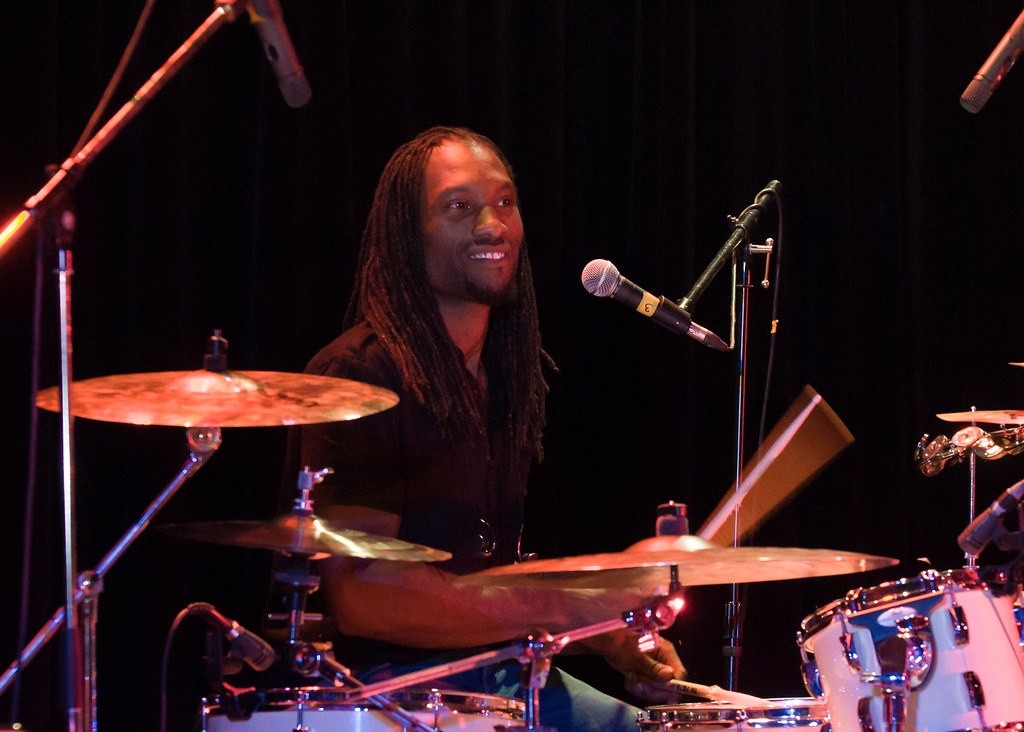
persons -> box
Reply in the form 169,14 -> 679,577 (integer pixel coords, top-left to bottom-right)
292,125 -> 685,732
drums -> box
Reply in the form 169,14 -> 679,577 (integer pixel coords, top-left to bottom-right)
797,564 -> 1024,732
196,683 -> 527,732
634,697 -> 829,732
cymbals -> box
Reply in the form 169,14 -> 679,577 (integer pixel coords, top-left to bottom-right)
915,422 -> 1024,476
935,410 -> 1024,426
34,367 -> 401,429
454,531 -> 902,591
169,514 -> 453,566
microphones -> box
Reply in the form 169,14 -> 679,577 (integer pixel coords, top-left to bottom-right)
960,10 -> 1024,114
957,480 -> 1024,555
245,0 -> 311,108
186,602 -> 275,672
580,259 -> 727,351
